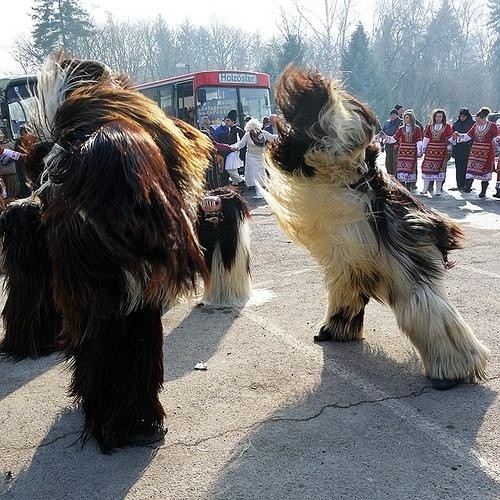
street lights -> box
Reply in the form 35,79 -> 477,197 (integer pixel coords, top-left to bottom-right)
175,61 -> 192,74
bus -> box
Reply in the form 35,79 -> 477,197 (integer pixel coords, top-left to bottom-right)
0,75 -> 39,145
121,70 -> 273,142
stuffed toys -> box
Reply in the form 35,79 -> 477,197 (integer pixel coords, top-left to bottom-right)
0,53 -> 277,457
255,61 -> 492,391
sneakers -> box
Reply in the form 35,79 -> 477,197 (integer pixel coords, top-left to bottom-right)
406,186 -> 500,198
231,180 -> 262,199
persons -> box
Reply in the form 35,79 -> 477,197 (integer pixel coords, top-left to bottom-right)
376,104 -> 500,202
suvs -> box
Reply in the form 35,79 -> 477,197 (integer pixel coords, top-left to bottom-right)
446,111 -> 500,162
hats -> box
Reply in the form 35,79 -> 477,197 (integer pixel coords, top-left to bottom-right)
395,104 -> 402,110
225,110 -> 238,122
389,109 -> 400,116
